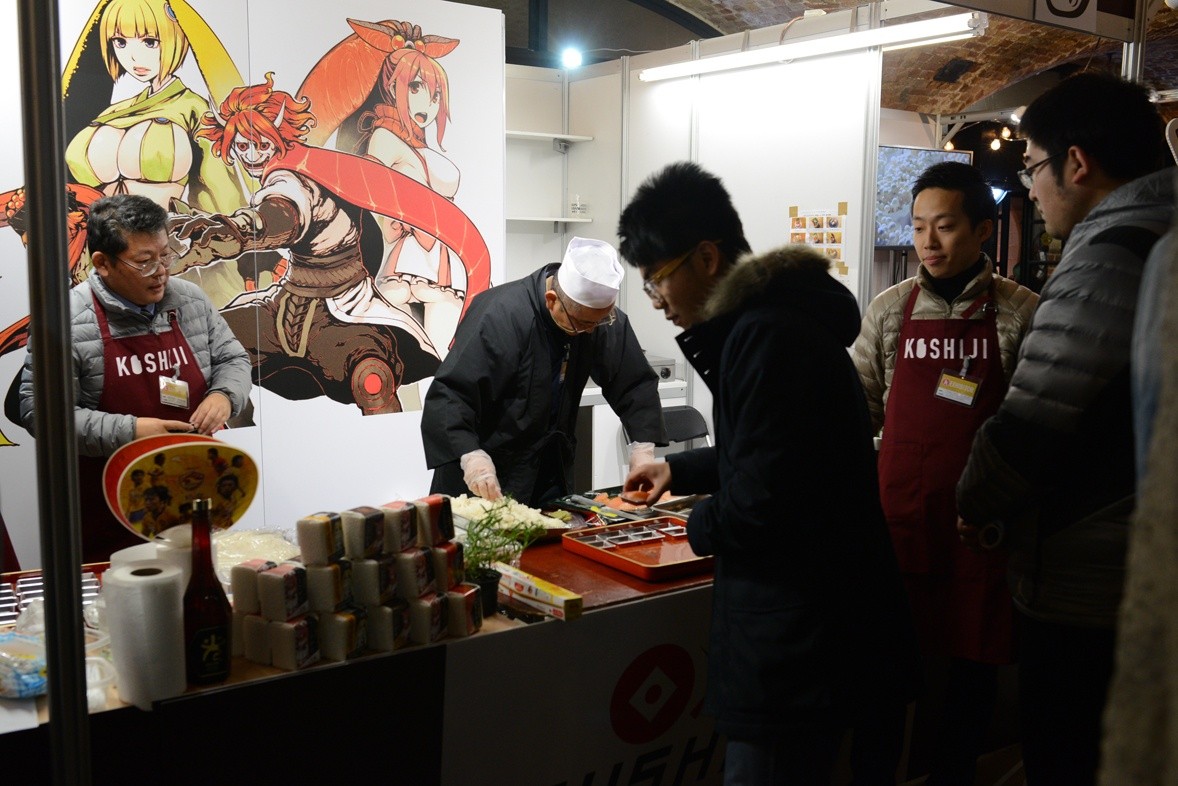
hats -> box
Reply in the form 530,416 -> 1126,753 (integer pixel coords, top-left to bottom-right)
558,236 -> 626,310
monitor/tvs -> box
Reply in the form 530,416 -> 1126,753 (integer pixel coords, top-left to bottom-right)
873,142 -> 974,250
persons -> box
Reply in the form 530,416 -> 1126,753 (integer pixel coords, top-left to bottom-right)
1101,230 -> 1178,785
24,194 -> 254,560
616,160 -> 882,785
952,72 -> 1177,784
848,161 -> 1042,784
422,235 -> 670,500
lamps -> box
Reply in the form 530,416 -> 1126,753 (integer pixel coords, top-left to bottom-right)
639,8 -> 990,84
1011,104 -> 1028,122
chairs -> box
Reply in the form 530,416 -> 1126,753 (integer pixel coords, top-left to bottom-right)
625,406 -> 713,460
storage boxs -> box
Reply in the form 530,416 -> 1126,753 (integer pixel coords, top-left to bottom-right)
490,561 -> 583,623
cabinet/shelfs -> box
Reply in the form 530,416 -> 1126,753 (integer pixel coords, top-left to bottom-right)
505,127 -> 594,226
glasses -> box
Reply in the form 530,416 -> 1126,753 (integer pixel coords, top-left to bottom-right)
642,237 -> 720,303
114,245 -> 181,278
550,287 -> 617,335
1016,147 -> 1068,190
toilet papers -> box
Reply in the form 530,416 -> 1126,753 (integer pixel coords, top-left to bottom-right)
100,559 -> 192,715
